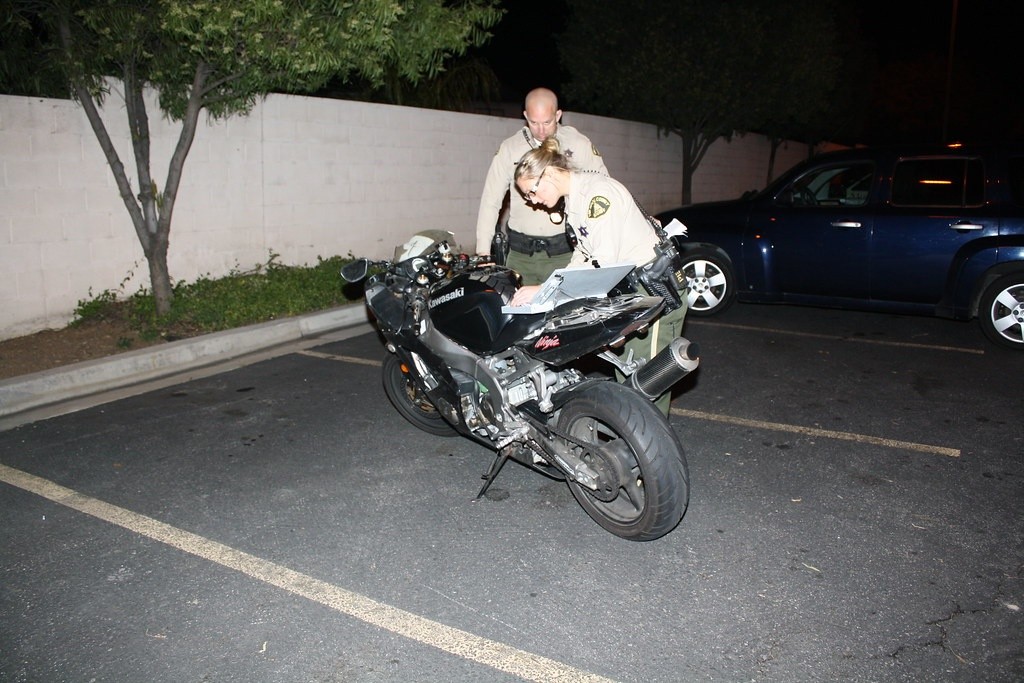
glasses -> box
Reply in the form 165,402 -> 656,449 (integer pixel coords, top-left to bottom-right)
524,168 -> 546,201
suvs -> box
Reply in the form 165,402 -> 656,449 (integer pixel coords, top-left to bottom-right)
654,145 -> 1024,350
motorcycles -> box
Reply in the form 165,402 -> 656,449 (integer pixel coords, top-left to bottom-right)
340,229 -> 702,543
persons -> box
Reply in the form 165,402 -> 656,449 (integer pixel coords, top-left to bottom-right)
513,136 -> 689,420
473,88 -> 611,286
